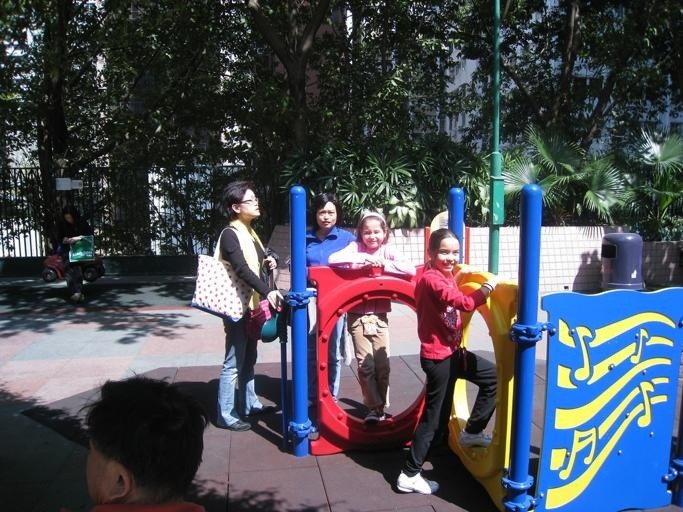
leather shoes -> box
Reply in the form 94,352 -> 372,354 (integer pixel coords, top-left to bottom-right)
216,407 -> 275,431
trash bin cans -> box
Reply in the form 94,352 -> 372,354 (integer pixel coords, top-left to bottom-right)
599,232 -> 645,290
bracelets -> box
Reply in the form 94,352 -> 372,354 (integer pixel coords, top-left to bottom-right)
482,282 -> 495,293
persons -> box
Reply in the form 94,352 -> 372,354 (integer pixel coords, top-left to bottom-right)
82,368 -> 206,512
59,204 -> 95,303
213,181 -> 279,431
284,193 -> 357,427
328,211 -> 416,422
395,229 -> 498,494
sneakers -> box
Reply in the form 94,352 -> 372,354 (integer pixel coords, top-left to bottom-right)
397,470 -> 439,494
364,409 -> 386,421
460,429 -> 491,448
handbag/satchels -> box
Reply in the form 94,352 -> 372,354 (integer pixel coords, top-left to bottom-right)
191,255 -> 272,341
